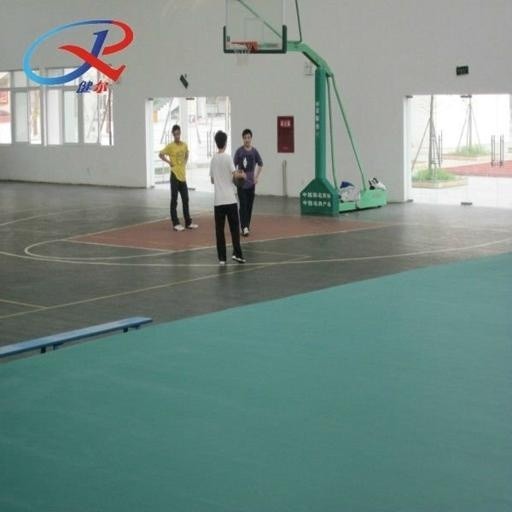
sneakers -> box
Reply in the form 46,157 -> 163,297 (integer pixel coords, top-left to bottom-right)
173,223 -> 198,231
232,253 -> 246,262
243,227 -> 249,235
219,261 -> 225,266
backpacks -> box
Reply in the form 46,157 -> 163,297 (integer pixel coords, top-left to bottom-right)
368,177 -> 386,190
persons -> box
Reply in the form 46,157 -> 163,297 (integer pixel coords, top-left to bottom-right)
159,124 -> 199,232
233,130 -> 263,236
210,132 -> 245,266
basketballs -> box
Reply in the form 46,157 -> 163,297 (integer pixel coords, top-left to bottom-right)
233,169 -> 246,187
